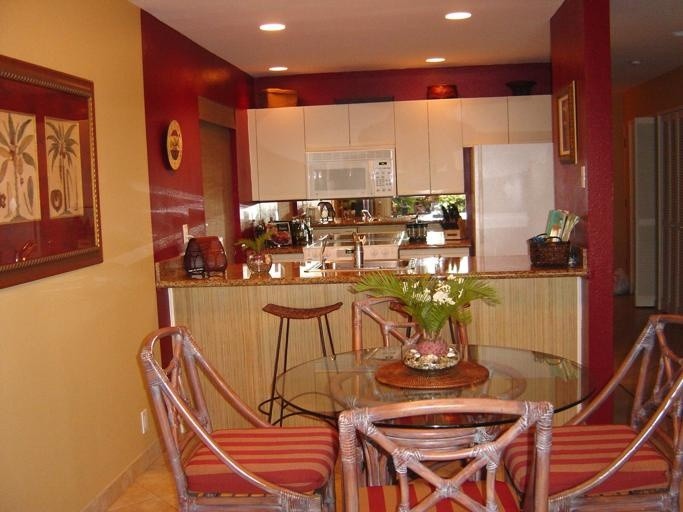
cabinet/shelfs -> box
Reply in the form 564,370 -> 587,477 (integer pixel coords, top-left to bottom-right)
399,240 -> 471,272
235,95 -> 552,202
293,217 -> 468,234
155,262 -> 587,429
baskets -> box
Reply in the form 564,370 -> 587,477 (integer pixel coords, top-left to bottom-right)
527,234 -> 570,268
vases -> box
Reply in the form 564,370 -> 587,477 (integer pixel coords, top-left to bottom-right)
416,335 -> 449,354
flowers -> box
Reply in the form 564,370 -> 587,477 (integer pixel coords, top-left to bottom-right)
350,265 -> 503,357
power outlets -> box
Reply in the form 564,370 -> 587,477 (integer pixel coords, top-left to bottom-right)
140,410 -> 150,434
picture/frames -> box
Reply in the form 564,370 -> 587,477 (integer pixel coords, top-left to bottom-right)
0,55 -> 103,289
362,210 -> 371,218
558,80 -> 578,165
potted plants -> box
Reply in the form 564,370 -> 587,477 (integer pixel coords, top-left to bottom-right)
235,229 -> 272,273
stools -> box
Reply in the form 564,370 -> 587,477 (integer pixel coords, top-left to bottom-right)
260,301 -> 344,427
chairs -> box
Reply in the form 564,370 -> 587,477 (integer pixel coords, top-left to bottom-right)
502,314 -> 683,512
353,297 -> 470,350
338,398 -> 552,512
137,327 -> 339,512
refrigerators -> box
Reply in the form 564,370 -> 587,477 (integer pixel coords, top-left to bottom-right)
462,143 -> 557,258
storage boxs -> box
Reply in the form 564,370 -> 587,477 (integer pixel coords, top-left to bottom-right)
267,89 -> 296,108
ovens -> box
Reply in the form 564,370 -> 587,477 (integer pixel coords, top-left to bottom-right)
304,149 -> 397,199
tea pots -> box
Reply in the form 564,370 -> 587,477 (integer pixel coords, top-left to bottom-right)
291,221 -> 312,247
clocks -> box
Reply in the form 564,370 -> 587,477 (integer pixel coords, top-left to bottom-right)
167,120 -> 183,170
